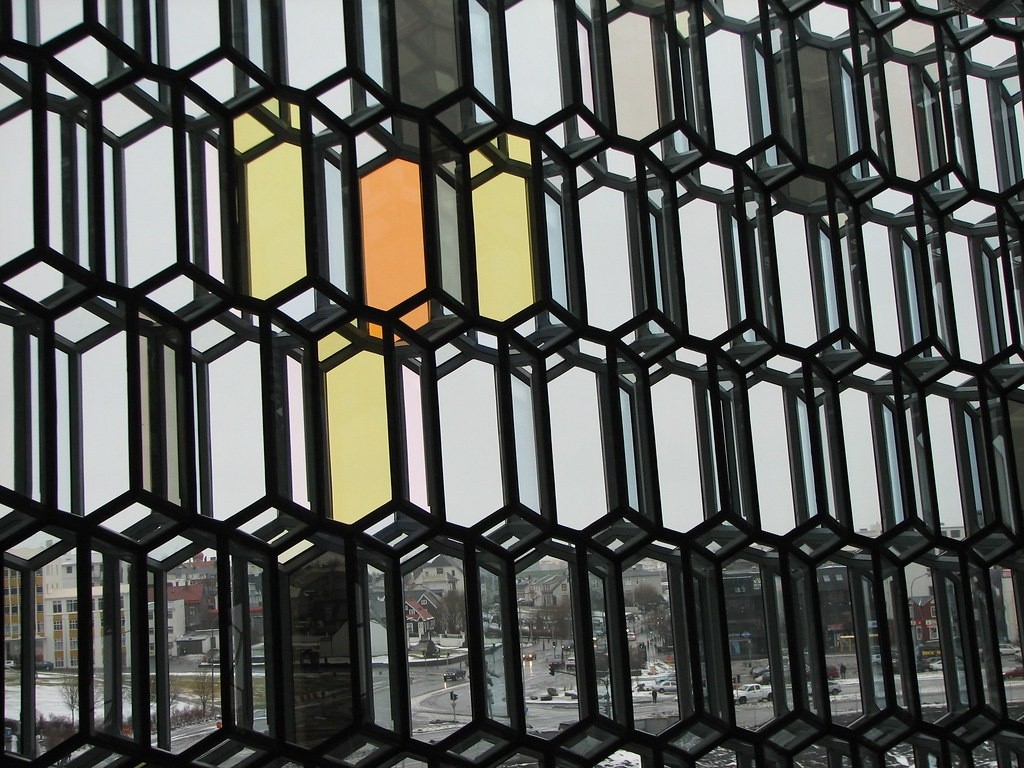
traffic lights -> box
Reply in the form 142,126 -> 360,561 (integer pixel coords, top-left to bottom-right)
450,691 -> 453,700
549,662 -> 554,675
916,615 -> 922,626
454,695 -> 458,701
737,675 -> 741,683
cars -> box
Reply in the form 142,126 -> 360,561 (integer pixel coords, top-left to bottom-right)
750,655 -> 792,684
872,654 -> 881,664
930,658 -> 961,671
523,650 -> 537,661
36,660 -> 54,672
655,681 -> 677,693
1003,666 -> 1024,679
5,660 -> 16,669
1014,652 -> 1023,662
808,681 -> 841,697
561,642 -> 573,651
628,632 -> 636,640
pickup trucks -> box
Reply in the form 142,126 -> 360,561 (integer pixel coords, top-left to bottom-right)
444,668 -> 466,681
733,683 -> 772,704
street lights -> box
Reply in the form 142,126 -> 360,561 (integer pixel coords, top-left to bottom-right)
208,624 -> 219,715
910,573 -> 932,651
647,625 -> 653,664
517,576 -> 532,638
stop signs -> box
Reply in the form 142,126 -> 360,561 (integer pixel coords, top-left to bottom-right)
123,726 -> 130,735
216,722 -> 221,728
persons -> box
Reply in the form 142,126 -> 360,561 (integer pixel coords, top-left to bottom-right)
652,689 -> 658,703
748,662 -> 752,668
216,722 -> 222,729
840,663 -> 847,679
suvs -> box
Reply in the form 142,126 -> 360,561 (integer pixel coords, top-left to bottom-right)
806,665 -> 840,681
999,643 -> 1021,655
565,658 -> 576,670
